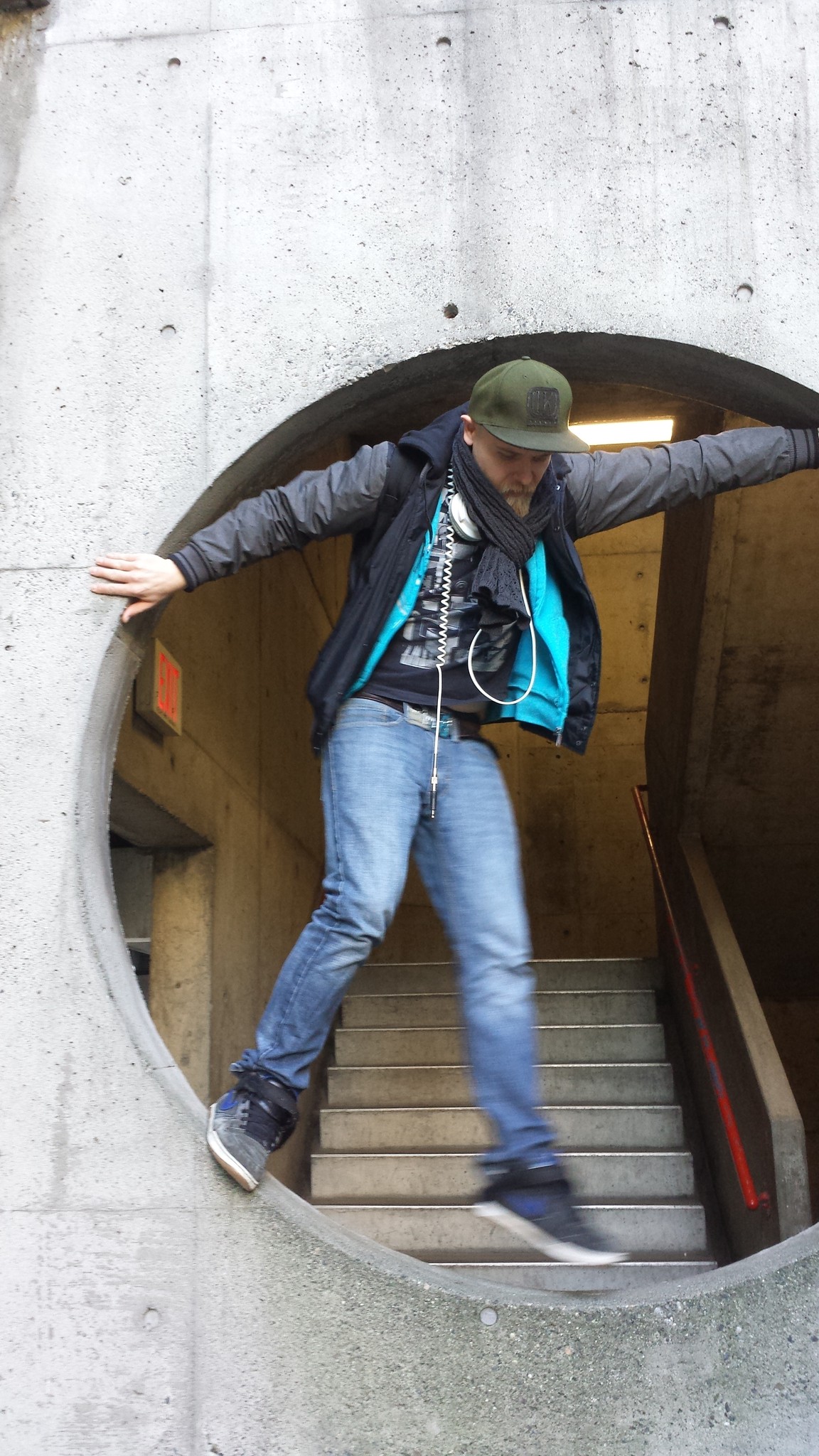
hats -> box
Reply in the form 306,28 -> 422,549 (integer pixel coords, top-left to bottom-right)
468,355 -> 590,455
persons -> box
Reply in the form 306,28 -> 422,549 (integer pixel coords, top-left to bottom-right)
86,353 -> 818,1264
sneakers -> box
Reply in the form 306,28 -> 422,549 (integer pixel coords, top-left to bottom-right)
474,1165 -> 628,1267
205,1073 -> 301,1191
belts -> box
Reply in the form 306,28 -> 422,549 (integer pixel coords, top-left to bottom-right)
352,690 -> 481,739
